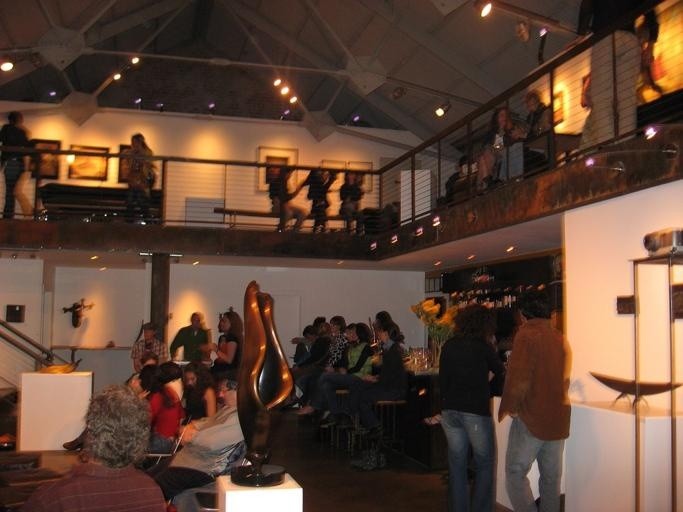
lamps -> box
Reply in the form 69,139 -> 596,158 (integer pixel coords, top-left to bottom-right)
433,99 -> 451,118
471,1 -> 551,45
582,144 -> 678,175
642,116 -> 682,142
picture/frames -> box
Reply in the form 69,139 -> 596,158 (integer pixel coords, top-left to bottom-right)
29,138 -> 60,180
321,158 -> 347,193
117,143 -> 133,184
68,144 -> 109,182
348,161 -> 373,192
257,145 -> 298,192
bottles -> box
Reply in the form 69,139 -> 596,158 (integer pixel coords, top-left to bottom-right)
450,284 -> 544,309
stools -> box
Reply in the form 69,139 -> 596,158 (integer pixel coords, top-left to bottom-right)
327,387 -> 409,459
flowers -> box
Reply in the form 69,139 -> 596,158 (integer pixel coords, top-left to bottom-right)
409,299 -> 462,343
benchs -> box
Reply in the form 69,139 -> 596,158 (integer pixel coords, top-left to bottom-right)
212,206 -> 374,234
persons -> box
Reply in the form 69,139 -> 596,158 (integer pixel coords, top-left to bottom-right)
123,133 -> 156,224
509,91 -> 551,165
269,167 -> 310,234
130,310 -> 246,501
307,171 -> 336,234
0,111 -> 31,218
340,172 -> 363,233
275,292 -> 569,512
578,0 -> 659,148
480,107 -> 515,188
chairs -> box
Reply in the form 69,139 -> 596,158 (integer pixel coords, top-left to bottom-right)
0,408 -> 255,512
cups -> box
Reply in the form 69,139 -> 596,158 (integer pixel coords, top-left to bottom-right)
407,344 -> 433,373
